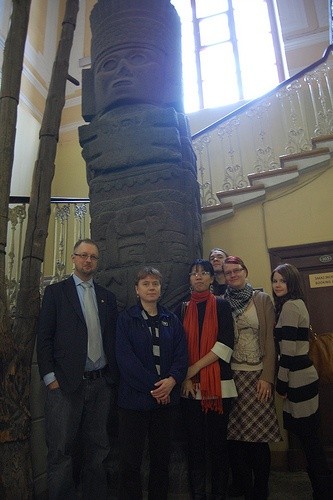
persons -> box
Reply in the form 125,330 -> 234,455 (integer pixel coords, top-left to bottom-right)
173,260 -> 238,500
209,249 -> 319,500
36,238 -> 119,500
115,269 -> 190,500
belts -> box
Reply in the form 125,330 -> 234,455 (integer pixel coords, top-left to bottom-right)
83,370 -> 101,380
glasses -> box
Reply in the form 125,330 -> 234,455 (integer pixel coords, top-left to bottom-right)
74,252 -> 100,261
189,271 -> 210,277
223,268 -> 244,275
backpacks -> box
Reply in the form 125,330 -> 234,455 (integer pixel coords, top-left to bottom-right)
305,327 -> 333,386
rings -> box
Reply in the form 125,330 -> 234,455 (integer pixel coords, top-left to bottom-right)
267,390 -> 269,393
